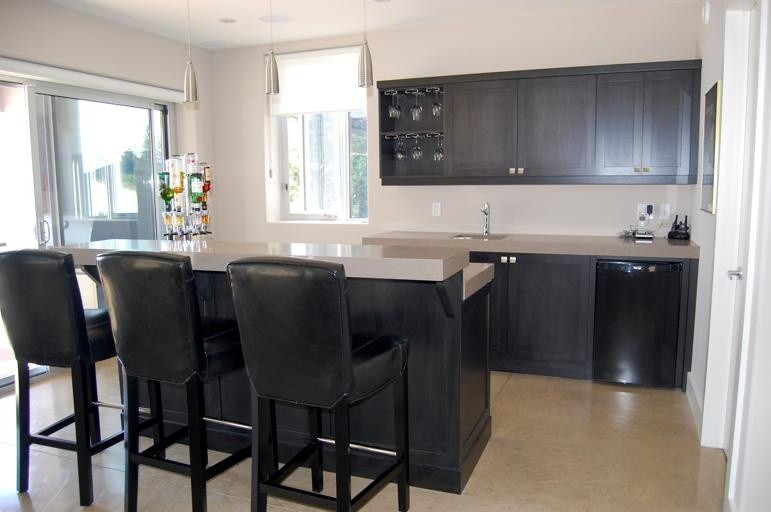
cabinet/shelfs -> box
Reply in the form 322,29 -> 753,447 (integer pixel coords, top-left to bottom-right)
447,65 -> 597,186
376,77 -> 447,187
469,252 -> 595,381
597,58 -> 703,186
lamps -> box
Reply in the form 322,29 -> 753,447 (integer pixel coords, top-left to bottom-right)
358,0 -> 373,88
184,1 -> 198,104
265,0 -> 280,95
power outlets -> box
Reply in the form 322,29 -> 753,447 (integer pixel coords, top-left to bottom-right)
637,203 -> 655,221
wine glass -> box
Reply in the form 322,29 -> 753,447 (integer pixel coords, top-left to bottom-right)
384,87 -> 444,161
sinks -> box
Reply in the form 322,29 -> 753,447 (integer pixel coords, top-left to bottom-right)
451,233 -> 506,241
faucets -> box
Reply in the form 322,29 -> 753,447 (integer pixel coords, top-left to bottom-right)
481,202 -> 490,235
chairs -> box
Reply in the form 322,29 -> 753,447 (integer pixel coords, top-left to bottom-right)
0,248 -> 125,506
96,249 -> 253,512
226,255 -> 410,512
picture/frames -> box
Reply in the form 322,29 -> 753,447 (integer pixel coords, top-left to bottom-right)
699,80 -> 722,215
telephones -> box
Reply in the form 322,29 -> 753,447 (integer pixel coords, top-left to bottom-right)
633,212 -> 654,239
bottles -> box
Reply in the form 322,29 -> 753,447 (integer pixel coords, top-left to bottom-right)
158,152 -> 212,241
637,212 -> 646,235
